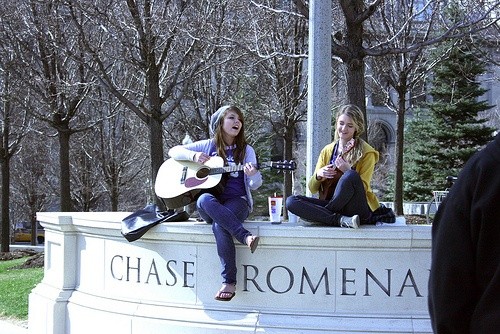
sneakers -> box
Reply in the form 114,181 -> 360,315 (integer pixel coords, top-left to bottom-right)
340,214 -> 360,229
299,217 -> 321,225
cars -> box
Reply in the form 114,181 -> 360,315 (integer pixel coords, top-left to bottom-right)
10,227 -> 45,244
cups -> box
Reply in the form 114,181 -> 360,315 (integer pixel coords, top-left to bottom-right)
267,196 -> 283,225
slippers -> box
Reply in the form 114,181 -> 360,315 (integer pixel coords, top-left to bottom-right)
249,236 -> 260,253
215,291 -> 235,300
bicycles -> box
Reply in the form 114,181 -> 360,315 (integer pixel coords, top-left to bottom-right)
426,176 -> 458,224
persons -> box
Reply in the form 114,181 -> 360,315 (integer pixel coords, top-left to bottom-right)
169,105 -> 264,301
285,104 -> 380,228
427,132 -> 500,334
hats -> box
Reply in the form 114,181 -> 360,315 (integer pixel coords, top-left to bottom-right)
210,105 -> 230,135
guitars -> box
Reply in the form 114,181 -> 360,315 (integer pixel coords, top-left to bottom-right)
154,155 -> 297,208
318,138 -> 356,202
